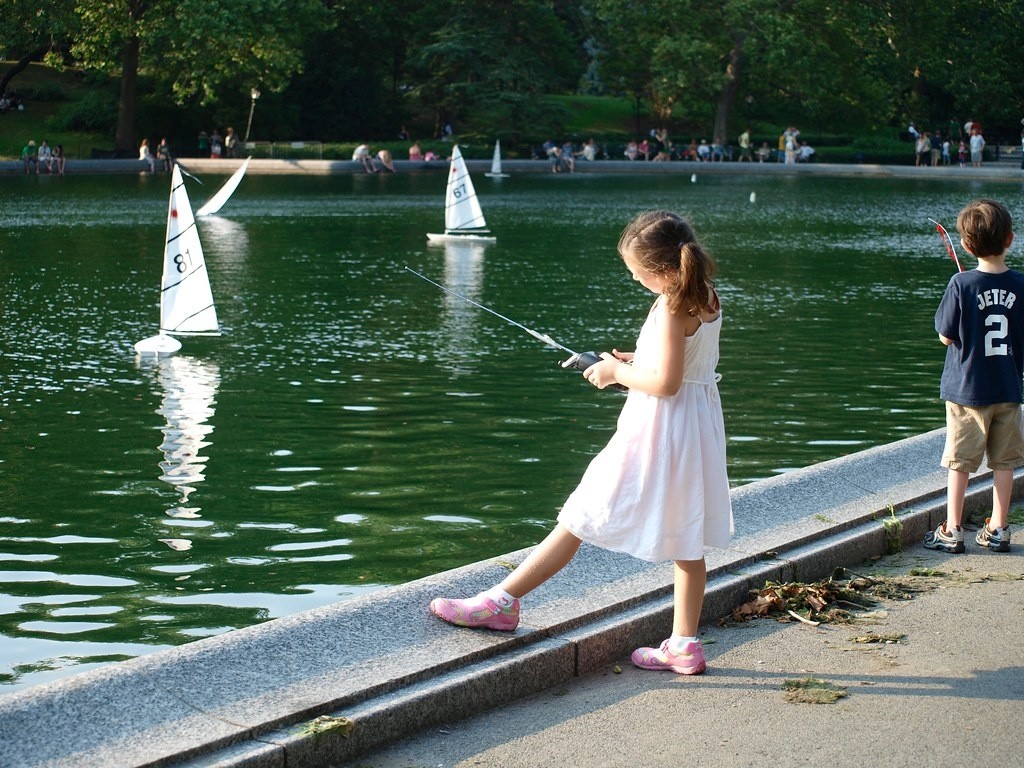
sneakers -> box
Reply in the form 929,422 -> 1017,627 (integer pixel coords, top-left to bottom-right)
630,638 -> 707,674
922,520 -> 966,554
975,517 -> 1011,552
430,591 -> 520,631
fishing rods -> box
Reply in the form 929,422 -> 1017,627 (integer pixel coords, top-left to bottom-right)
926,214 -> 964,275
404,264 -> 622,386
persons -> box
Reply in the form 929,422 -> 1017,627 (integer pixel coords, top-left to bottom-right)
400,124 -> 409,140
542,139 -> 599,173
375,150 -> 396,173
21,141 -> 39,176
52,145 -> 66,175
225,126 -> 241,158
923,198 -> 1024,554
624,126 -> 672,162
430,209 -> 734,675
139,139 -> 156,175
209,126 -> 223,159
353,140 -> 379,174
425,148 -> 440,162
39,139 -> 54,174
675,125 -> 815,163
157,138 -> 173,171
909,118 -> 985,167
410,140 -> 424,161
196,130 -> 211,157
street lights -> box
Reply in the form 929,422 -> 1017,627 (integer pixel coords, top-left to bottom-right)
242,85 -> 262,141
634,85 -> 644,141
744,92 -> 753,128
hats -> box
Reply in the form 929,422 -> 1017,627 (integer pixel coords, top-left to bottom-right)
28,141 -> 36,147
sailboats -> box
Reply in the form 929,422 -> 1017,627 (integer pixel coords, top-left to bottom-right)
426,143 -> 498,244
484,139 -> 512,179
133,164 -> 222,358
196,153 -> 255,222
